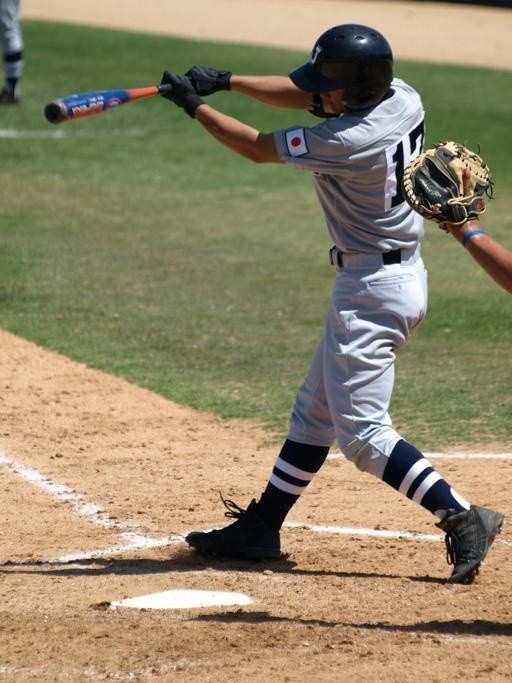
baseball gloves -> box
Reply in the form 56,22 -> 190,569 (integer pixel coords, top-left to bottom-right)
401,140 -> 490,225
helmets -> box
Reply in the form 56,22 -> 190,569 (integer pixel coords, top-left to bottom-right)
288,24 -> 394,110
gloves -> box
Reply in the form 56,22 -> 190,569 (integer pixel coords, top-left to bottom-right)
184,65 -> 231,96
159,71 -> 206,118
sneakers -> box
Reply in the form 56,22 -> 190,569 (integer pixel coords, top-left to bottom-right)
186,492 -> 281,559
434,504 -> 504,584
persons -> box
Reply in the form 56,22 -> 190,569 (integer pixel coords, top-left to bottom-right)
157,22 -> 506,586
404,139 -> 512,305
0,0 -> 26,103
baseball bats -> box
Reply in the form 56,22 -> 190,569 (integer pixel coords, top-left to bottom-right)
43,86 -> 176,125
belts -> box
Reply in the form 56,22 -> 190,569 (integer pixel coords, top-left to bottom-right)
328,246 -> 400,267
